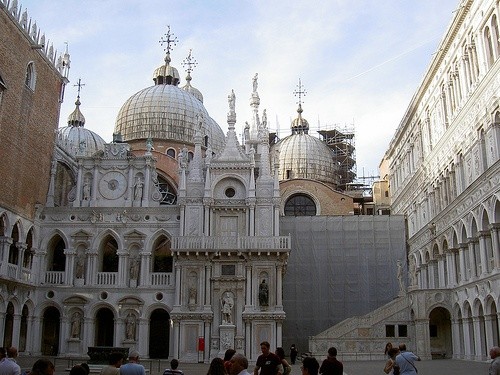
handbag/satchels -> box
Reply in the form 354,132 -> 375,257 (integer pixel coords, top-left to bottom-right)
415,368 -> 417,373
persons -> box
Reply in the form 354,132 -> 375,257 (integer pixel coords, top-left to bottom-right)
252,73 -> 259,95
262,109 -> 268,128
0,341 -> 343,375
228,89 -> 236,115
125,312 -> 135,338
75,250 -> 86,279
488,347 -> 500,375
71,312 -> 81,338
131,177 -> 144,206
2,0 -> 71,78
221,292 -> 233,324
177,145 -> 188,167
205,146 -> 213,160
243,122 -> 251,139
83,180 -> 91,201
383,342 -> 421,375
189,283 -> 198,304
260,279 -> 268,306
130,255 -> 140,279
250,146 -> 256,164
